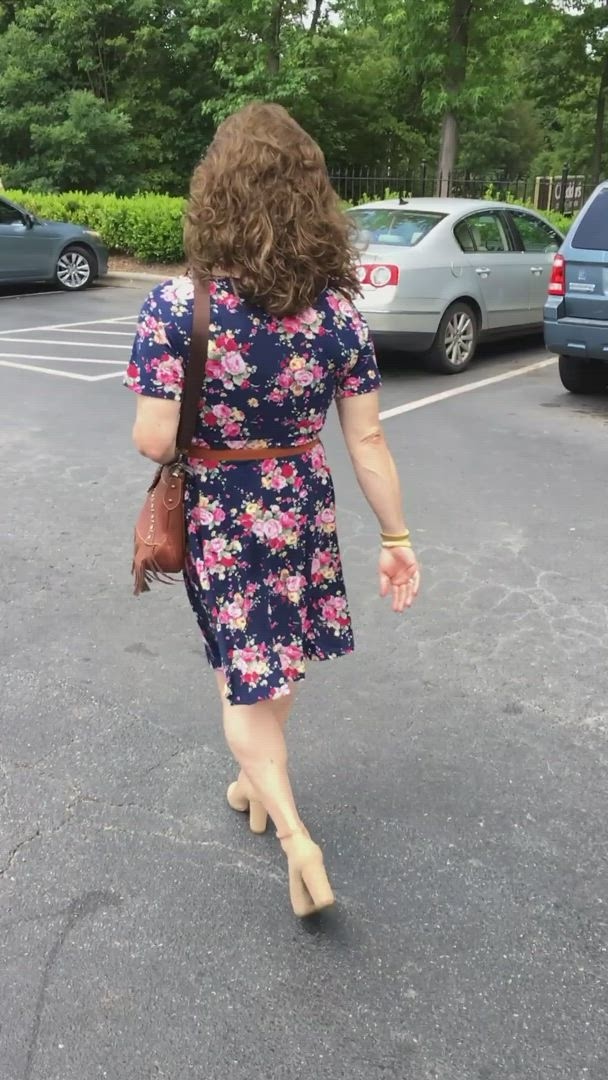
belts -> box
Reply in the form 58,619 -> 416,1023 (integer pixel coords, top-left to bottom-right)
192,434 -> 322,461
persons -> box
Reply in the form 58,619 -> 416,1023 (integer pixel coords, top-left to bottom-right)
123,104 -> 420,918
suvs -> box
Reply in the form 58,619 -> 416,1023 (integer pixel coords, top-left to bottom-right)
540,177 -> 608,398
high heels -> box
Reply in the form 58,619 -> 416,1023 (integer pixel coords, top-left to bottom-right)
226,774 -> 269,832
287,844 -> 334,918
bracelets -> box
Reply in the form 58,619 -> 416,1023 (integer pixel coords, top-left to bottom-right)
380,529 -> 411,548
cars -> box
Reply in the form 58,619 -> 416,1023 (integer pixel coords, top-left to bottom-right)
330,193 -> 567,377
0,194 -> 109,293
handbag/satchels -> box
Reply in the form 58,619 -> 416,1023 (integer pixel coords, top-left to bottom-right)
131,463 -> 187,595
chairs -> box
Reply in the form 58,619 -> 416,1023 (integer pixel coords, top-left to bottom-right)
457,223 -> 487,251
